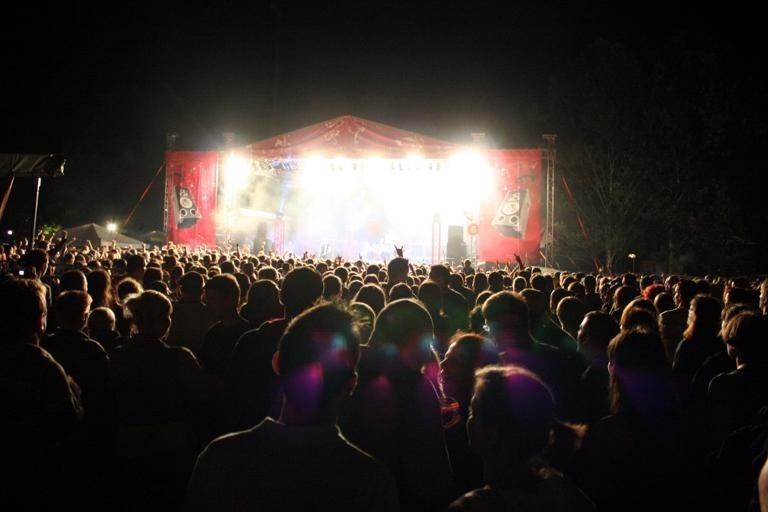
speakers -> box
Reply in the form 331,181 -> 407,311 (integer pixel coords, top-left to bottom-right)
173,187 -> 202,229
492,189 -> 532,239
448,226 -> 466,266
253,222 -> 271,254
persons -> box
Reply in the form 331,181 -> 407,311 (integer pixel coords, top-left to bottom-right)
0,232 -> 768,512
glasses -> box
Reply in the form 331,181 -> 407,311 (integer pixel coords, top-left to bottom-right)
482,321 -> 494,332
